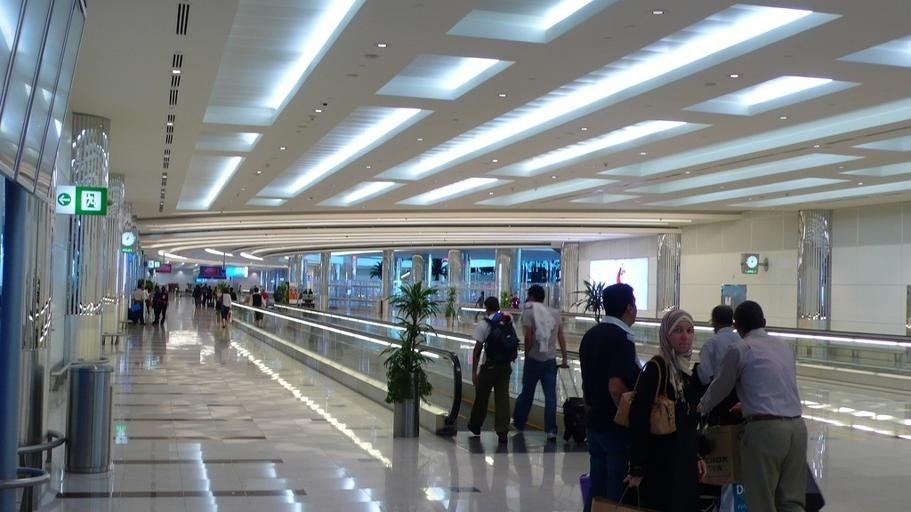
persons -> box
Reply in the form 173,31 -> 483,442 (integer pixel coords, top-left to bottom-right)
192,283 -> 269,328
468,295 -> 516,444
510,285 -> 569,441
133,281 -> 168,325
476,292 -> 484,308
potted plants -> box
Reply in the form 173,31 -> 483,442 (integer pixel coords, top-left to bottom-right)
383,281 -> 446,437
442,287 -> 458,328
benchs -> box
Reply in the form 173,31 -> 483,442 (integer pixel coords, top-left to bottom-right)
101,330 -> 122,345
121,319 -> 134,330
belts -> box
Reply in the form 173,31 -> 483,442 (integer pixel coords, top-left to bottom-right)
752,413 -> 801,420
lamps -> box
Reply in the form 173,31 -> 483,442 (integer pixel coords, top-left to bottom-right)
740,252 -> 770,274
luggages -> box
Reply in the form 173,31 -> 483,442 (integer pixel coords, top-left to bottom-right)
556,364 -> 588,443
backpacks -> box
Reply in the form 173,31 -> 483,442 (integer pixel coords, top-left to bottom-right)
483,315 -> 519,363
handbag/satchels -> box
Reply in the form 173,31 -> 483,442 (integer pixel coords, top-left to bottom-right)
216,301 -> 224,310
590,485 -> 645,512
129,303 -> 142,321
615,354 -> 677,435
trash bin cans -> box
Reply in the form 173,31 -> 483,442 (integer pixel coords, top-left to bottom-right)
65,364 -> 114,474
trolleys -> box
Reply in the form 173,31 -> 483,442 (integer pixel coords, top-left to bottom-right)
297,293 -> 314,310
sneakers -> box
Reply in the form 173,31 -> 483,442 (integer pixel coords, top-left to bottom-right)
510,417 -> 522,432
499,433 -> 508,442
546,432 -> 556,440
467,422 -> 480,435
